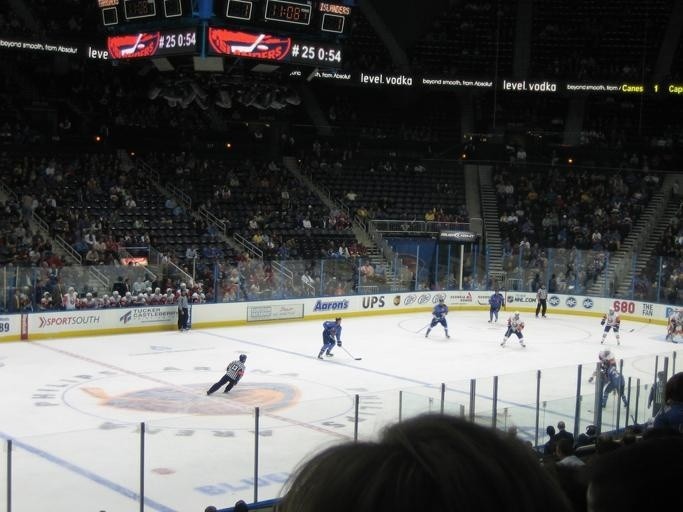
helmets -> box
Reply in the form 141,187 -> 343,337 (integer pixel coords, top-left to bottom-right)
239,354 -> 247,362
18,282 -> 206,300
336,316 -> 341,321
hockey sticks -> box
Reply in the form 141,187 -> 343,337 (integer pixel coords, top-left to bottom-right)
330,335 -> 362,360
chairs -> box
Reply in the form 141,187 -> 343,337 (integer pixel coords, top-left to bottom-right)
0,1 -> 683,317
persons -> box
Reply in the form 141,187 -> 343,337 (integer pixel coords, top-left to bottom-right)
1,76 -> 682,512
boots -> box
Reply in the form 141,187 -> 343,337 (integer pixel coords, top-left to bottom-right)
318,353 -> 324,359
325,348 -> 333,356
